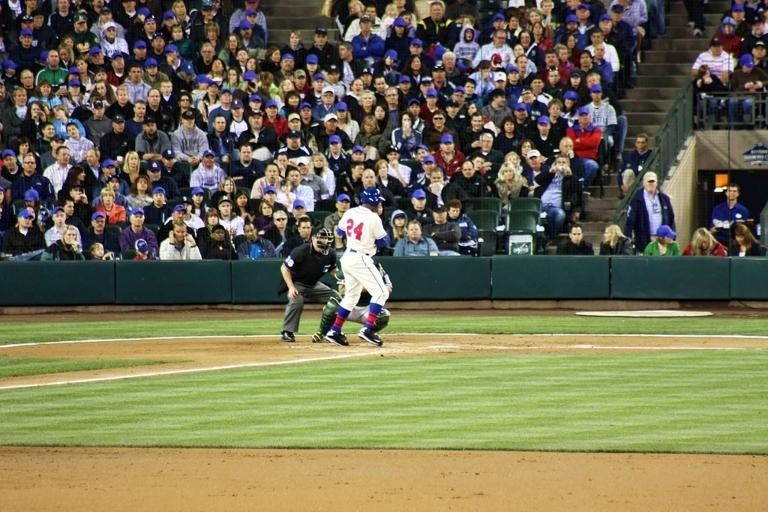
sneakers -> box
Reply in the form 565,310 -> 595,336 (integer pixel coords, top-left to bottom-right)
283,331 -> 294,342
313,332 -> 323,343
358,327 -> 383,346
325,327 -> 349,346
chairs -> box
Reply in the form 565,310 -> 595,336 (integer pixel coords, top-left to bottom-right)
146,143 -> 561,267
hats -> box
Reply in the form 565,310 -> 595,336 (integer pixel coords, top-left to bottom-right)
1,2 -> 624,220
656,225 -> 676,237
644,171 -> 658,183
710,4 -> 765,67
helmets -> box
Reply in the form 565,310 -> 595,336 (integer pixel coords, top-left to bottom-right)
311,226 -> 334,256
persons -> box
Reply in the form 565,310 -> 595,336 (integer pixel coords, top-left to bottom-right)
2,3 -> 766,263
277,185 -> 395,349
